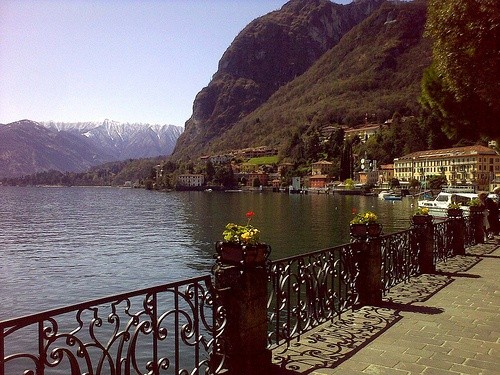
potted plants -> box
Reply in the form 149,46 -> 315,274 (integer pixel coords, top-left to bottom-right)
468,198 -> 485,211
447,202 -> 463,216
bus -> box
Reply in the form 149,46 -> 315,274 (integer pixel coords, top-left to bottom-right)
442,186 -> 475,193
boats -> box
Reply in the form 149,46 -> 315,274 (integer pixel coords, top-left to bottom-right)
383,194 -> 403,200
378,191 -> 390,200
365,192 -> 374,196
204,189 -> 213,192
417,192 -> 498,220
225,189 -> 242,193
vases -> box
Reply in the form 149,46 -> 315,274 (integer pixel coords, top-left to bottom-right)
409,214 -> 434,225
215,240 -> 271,266
349,221 -> 383,238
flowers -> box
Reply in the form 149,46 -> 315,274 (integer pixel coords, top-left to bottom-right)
414,205 -> 429,216
224,211 -> 261,243
348,209 -> 377,224
